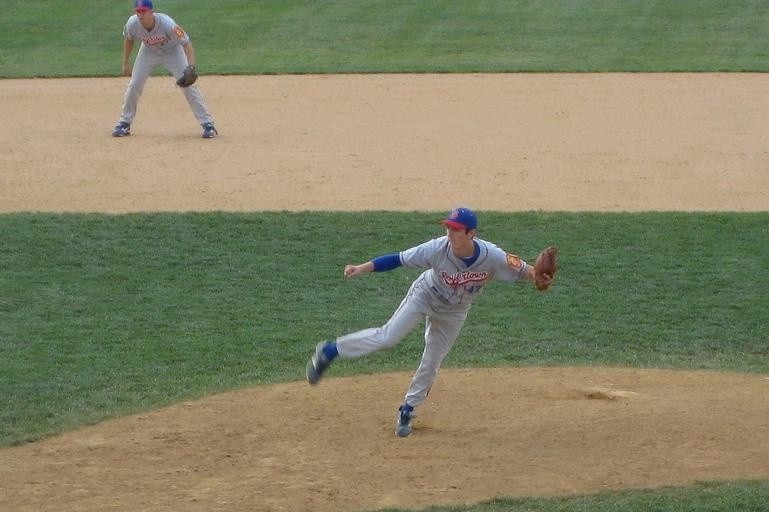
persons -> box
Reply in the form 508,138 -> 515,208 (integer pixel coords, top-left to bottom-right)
304,207 -> 559,441
111,0 -> 221,142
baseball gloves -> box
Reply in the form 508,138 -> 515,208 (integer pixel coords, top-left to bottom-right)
534,246 -> 558,291
176,65 -> 197,87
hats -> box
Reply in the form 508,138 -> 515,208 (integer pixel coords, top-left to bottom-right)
438,207 -> 478,232
133,1 -> 153,13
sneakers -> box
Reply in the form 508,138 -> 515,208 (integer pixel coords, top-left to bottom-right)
111,122 -> 131,136
394,404 -> 417,439
200,123 -> 218,138
306,339 -> 334,387
